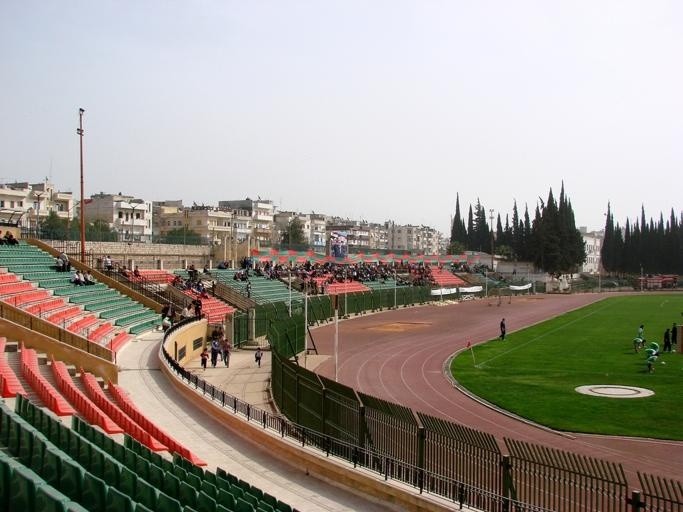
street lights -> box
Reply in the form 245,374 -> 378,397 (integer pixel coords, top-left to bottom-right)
123,200 -> 143,244
55,203 -> 79,240
28,187 -> 49,239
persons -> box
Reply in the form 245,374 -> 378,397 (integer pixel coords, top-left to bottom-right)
497,318 -> 506,341
633,322 -> 677,373
448,262 -> 494,274
55,252 -> 71,272
71,271 -> 96,286
134,265 -> 141,277
121,266 -> 130,277
161,256 -> 443,370
106,256 -> 112,270
0,230 -> 19,244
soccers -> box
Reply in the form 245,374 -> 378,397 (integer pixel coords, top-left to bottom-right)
672,350 -> 676,353
661,362 -> 665,365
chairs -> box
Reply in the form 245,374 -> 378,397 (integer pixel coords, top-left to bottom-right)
1,239 -> 302,512
280,261 -> 503,297
